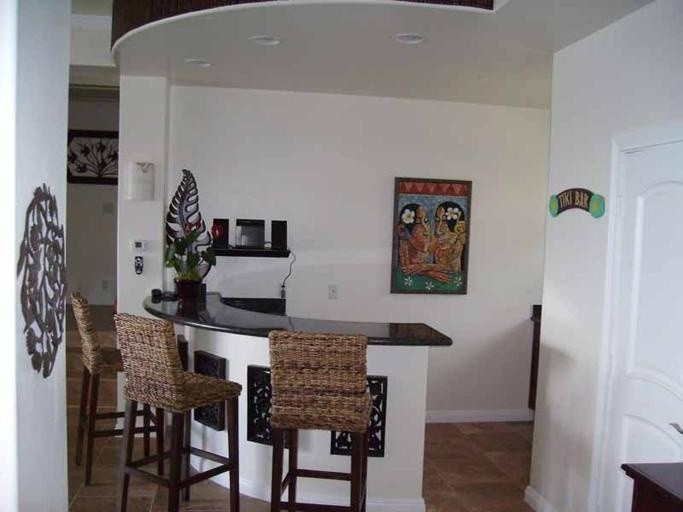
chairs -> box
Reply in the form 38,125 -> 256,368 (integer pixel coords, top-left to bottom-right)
66,286 -> 242,512
262,328 -> 374,511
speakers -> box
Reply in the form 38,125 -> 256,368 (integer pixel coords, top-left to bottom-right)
272,221 -> 287,250
213,219 -> 228,249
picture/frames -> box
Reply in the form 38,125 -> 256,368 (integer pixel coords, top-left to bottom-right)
66,129 -> 119,185
388,174 -> 473,296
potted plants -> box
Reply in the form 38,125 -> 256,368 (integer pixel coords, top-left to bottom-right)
161,168 -> 218,317
